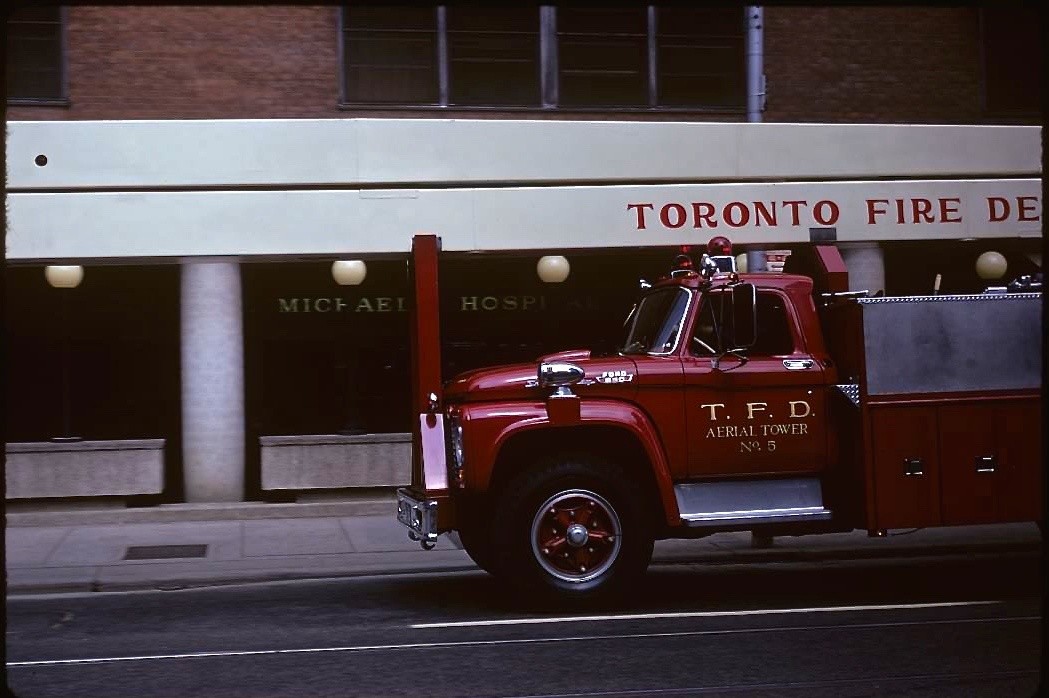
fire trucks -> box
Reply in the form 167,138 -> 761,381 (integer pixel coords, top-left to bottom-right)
394,232 -> 1048,617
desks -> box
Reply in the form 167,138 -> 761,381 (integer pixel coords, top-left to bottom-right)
259,434 -> 414,502
4,438 -> 167,507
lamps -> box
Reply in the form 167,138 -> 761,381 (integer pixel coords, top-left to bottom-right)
331,260 -> 366,286
536,255 -> 570,283
46,264 -> 83,288
976,250 -> 1009,280
